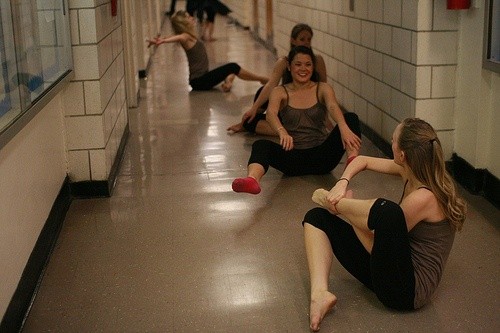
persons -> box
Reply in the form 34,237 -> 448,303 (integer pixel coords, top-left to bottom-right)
227,24 -> 336,135
145,10 -> 271,92
198,0 -> 218,42
165,0 -> 246,23
231,45 -> 363,196
300,119 -> 470,332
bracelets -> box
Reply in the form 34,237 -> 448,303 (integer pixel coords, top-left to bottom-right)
337,178 -> 350,187
